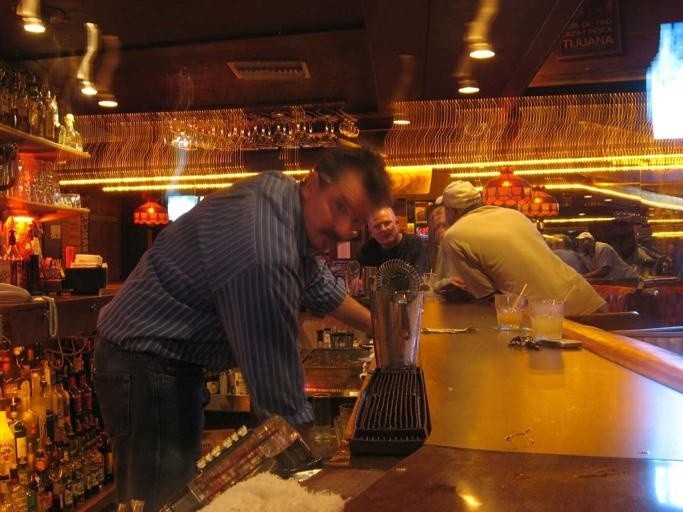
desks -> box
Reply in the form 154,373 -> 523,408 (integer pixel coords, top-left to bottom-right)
0,281 -> 126,345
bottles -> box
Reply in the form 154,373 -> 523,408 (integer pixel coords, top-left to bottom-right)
0,228 -> 28,291
0,61 -> 84,153
25,232 -> 43,295
0,358 -> 117,512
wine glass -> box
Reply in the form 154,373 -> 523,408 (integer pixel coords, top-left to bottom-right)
162,110 -> 360,151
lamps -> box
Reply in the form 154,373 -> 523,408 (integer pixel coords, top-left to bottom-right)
132,199 -> 169,227
482,166 -> 559,217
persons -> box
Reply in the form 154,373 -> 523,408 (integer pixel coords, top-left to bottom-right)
354,200 -> 430,279
428,180 -> 682,331
87,142 -> 396,510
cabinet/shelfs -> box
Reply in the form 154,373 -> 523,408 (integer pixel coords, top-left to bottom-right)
0,120 -> 91,221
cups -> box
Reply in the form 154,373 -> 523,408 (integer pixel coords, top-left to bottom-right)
493,293 -> 524,331
361,266 -> 377,298
526,297 -> 564,342
343,269 -> 360,297
369,288 -> 423,373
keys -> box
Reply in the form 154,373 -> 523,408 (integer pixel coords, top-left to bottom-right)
505,334 -> 543,351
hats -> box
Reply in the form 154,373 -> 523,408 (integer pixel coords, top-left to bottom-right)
435,180 -> 481,209
576,232 -> 595,243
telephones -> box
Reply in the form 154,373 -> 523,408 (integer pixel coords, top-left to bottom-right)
0,283 -> 31,302
